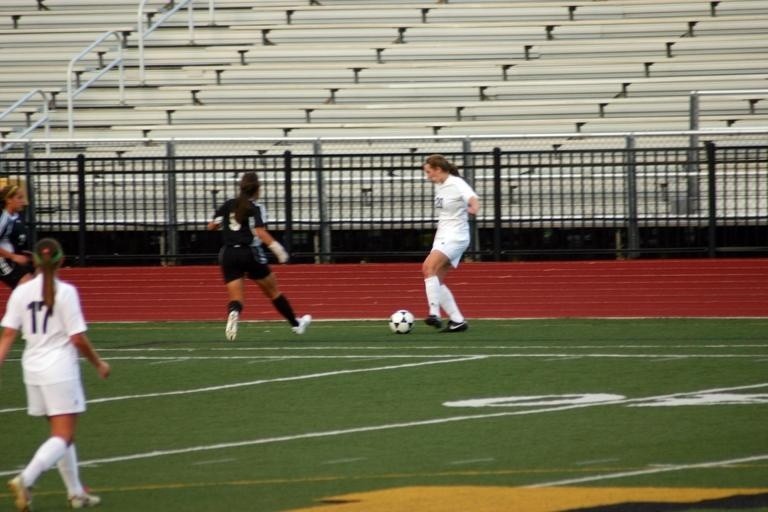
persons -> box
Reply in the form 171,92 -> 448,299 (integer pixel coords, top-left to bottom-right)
0,184 -> 38,289
1,237 -> 111,511
421,155 -> 481,334
206,171 -> 313,342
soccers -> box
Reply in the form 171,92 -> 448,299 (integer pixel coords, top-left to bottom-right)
390,310 -> 415,334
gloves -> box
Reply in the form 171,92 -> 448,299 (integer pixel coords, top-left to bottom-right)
268,241 -> 289,263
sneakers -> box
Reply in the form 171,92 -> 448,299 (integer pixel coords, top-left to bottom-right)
225,311 -> 240,342
7,475 -> 33,512
67,486 -> 101,509
425,315 -> 442,328
439,320 -> 468,333
291,314 -> 312,335
